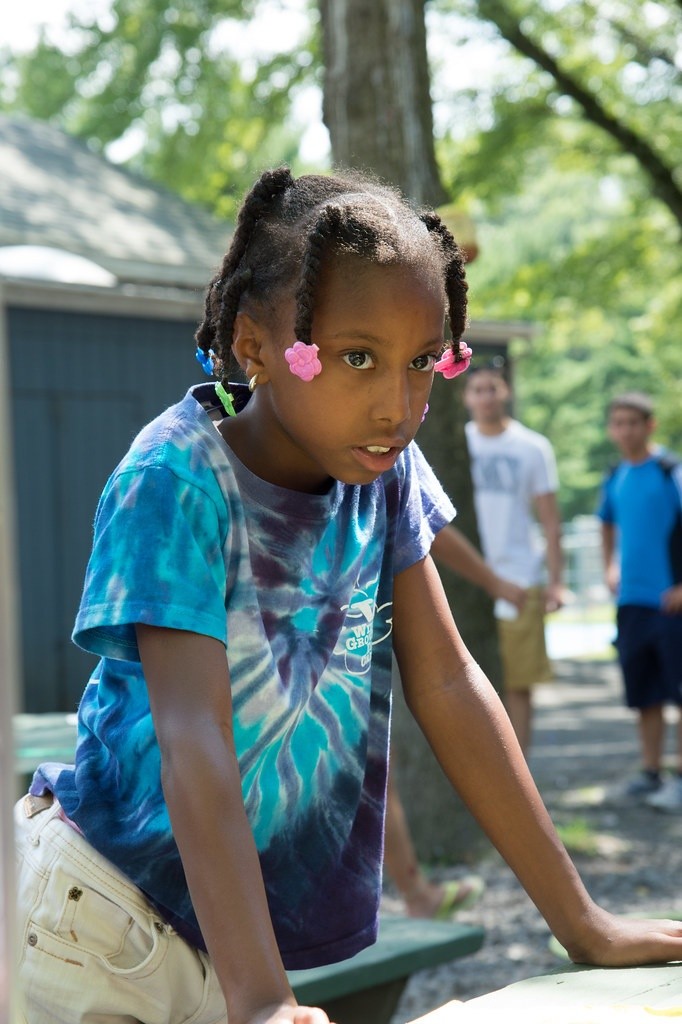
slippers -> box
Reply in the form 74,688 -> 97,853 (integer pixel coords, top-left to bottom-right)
437,874 -> 484,921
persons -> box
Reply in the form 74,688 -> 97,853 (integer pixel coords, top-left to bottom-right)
385,437 -> 527,921
460,359 -> 568,774
594,394 -> 681,799
12,166 -> 681,1022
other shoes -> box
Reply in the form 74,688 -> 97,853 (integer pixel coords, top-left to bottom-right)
646,778 -> 682,810
603,773 -> 663,806
